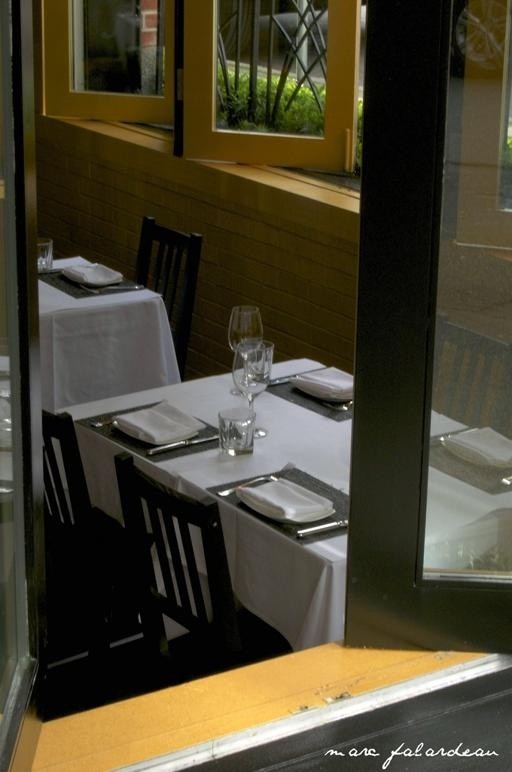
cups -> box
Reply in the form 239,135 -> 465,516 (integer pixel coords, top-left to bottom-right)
37,237 -> 54,274
216,407 -> 255,456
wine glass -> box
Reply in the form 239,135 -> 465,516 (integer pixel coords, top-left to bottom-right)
226,304 -> 275,440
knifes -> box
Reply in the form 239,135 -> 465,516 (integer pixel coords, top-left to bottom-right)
267,364 -> 326,386
147,434 -> 219,455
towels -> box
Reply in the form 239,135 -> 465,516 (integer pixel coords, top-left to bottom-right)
62,262 -> 124,285
442,425 -> 512,468
290,366 -> 354,400
111,399 -> 207,446
236,477 -> 333,522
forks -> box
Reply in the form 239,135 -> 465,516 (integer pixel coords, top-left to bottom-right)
77,284 -> 146,294
322,399 -> 353,412
216,461 -> 296,498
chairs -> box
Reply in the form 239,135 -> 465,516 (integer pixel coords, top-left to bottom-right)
134,216 -> 203,381
432,318 -> 512,439
114,451 -> 293,686
42,408 -> 142,708
